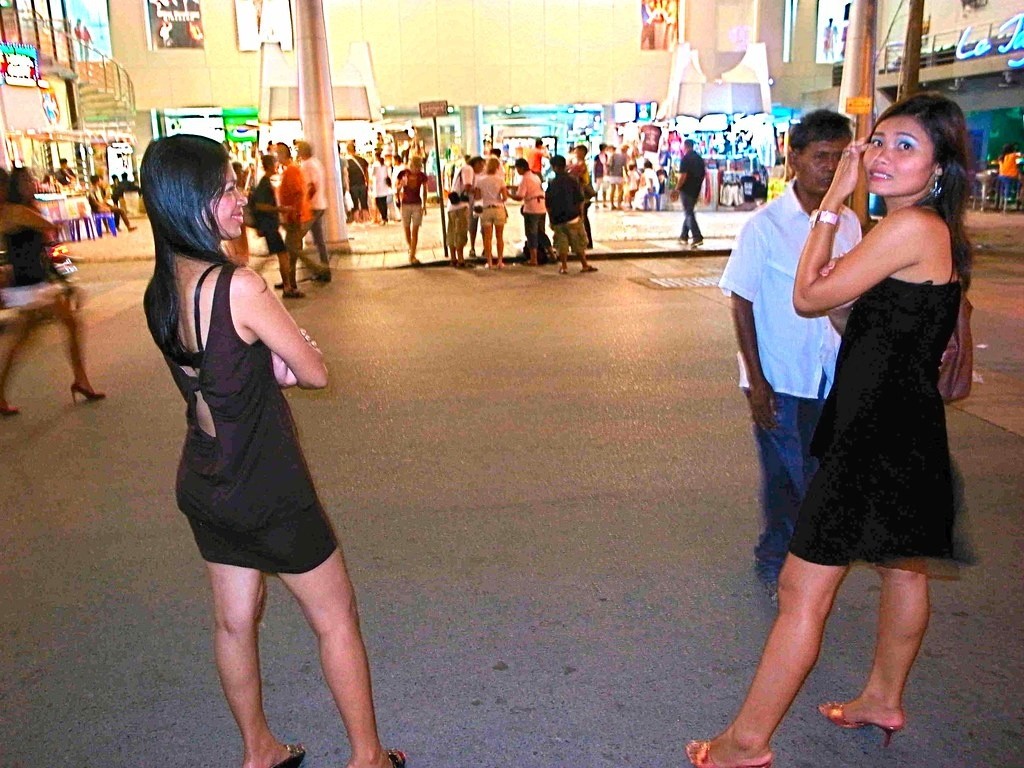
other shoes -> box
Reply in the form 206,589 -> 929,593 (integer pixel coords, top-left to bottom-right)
309,273 -> 332,282
282,289 -> 306,298
580,266 -> 598,272
275,282 -> 297,290
270,744 -> 305,768
385,748 -> 406,768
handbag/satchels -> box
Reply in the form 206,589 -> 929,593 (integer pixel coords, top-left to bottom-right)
938,293 -> 974,403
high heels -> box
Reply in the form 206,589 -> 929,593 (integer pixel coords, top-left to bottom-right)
686,740 -> 772,768
0,400 -> 19,414
819,701 -> 904,747
71,384 -> 105,404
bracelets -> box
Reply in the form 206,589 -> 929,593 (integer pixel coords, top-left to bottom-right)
808,209 -> 839,226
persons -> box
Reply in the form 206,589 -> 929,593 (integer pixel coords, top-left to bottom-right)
222,140 -> 707,300
157,17 -> 203,48
0,164 -> 107,417
996,142 -> 1024,204
641,0 -> 678,51
823,18 -> 838,61
682,95 -> 974,768
74,18 -> 94,62
140,134 -> 408,768
42,157 -> 142,233
